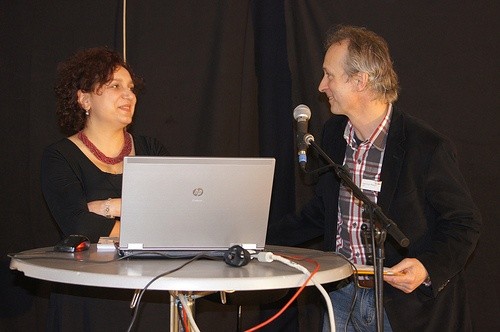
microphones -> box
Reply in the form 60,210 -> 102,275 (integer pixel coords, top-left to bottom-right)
293,104 -> 311,169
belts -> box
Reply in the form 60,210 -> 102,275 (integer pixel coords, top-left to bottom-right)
355,279 -> 376,290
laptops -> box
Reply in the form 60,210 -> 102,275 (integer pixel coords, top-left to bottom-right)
119,156 -> 276,258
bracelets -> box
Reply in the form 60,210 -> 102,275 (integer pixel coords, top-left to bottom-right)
105,198 -> 113,218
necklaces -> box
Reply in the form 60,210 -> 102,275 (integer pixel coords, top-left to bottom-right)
77,132 -> 133,165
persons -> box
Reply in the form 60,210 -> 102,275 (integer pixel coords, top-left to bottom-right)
265,26 -> 484,332
40,45 -> 171,332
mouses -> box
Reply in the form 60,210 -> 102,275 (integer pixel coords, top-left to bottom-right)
54,235 -> 91,252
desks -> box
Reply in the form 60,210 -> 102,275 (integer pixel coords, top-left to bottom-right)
10,243 -> 354,332
